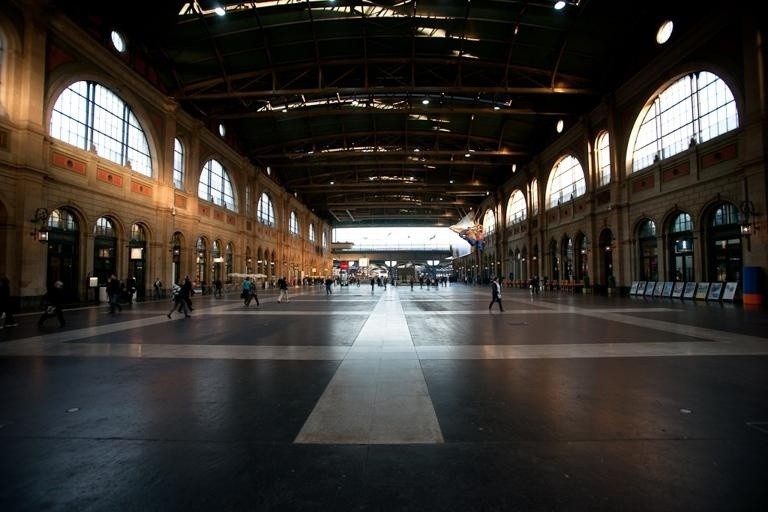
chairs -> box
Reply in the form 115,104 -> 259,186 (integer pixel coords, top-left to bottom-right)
503,279 -> 583,295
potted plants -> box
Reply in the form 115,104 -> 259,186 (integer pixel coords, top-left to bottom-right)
582,276 -> 591,294
607,275 -> 615,294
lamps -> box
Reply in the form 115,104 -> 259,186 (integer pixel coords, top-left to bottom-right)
245,256 -> 251,264
169,238 -> 180,255
31,208 -> 50,241
739,201 -> 760,251
456,237 -> 615,273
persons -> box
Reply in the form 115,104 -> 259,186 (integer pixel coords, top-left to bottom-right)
1,275 -> 138,329
371,275 -> 456,293
464,276 -> 538,311
272,276 -> 360,304
154,276 -> 260,319
